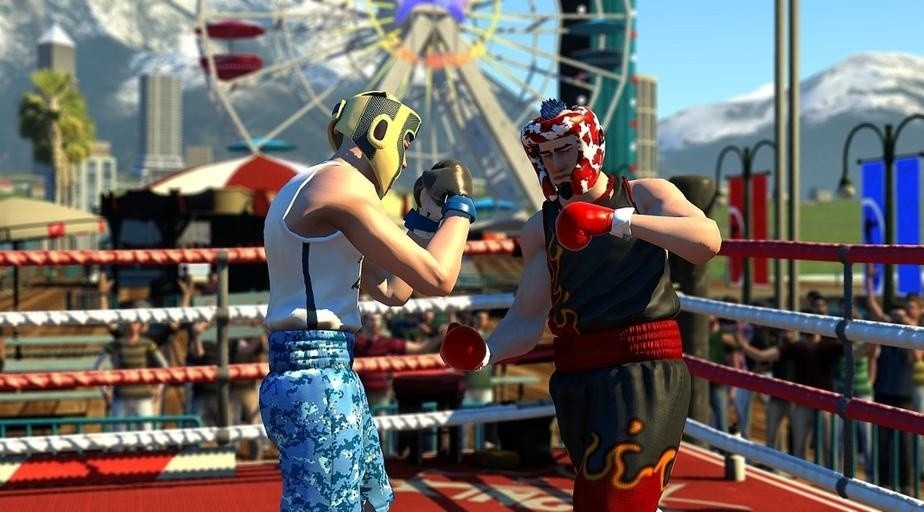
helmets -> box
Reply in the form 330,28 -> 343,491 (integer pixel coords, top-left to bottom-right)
520,106 -> 607,207
327,88 -> 424,201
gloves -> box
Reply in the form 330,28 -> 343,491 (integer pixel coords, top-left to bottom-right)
555,199 -> 635,252
403,158 -> 478,241
440,319 -> 492,374
